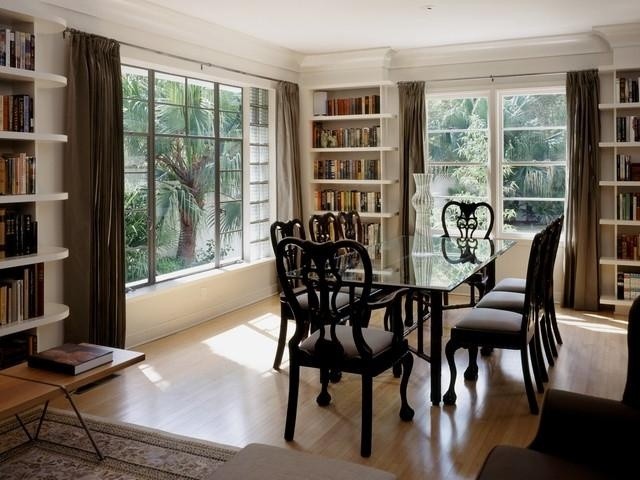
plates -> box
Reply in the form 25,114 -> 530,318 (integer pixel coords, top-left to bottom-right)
306,79 -> 402,294
599,64 -> 640,317
0,0 -> 72,370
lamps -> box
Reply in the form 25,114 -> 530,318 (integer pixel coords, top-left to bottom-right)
274,237 -> 419,455
444,212 -> 566,415
268,219 -> 365,383
337,209 -> 430,326
441,238 -> 494,265
309,210 -> 397,374
417,201 -> 494,322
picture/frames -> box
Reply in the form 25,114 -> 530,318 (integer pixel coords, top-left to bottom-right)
285,238 -> 537,409
1,344 -> 147,463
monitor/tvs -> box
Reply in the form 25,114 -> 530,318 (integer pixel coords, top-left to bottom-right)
312,95 -> 381,284
1,25 -> 46,373
28,342 -> 113,376
616,75 -> 640,302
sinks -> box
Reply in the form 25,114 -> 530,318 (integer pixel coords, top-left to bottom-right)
474,292 -> 639,479
201,442 -> 397,479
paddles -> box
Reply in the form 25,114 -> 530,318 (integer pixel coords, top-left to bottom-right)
0,406 -> 243,479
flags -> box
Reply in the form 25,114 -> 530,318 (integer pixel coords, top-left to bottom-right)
410,172 -> 436,233
411,235 -> 432,287
